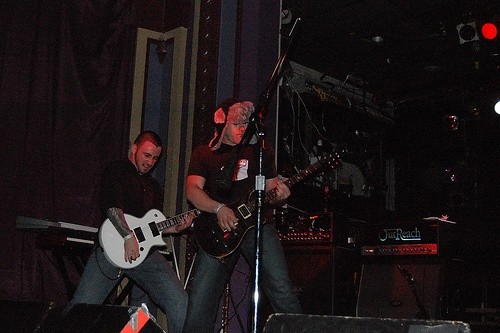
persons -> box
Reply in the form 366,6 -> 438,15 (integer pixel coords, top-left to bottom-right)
182,99 -> 302,333
63,131 -> 193,333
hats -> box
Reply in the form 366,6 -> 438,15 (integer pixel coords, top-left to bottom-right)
209,98 -> 259,150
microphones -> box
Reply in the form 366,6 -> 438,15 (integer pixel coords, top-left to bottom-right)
224,147 -> 243,181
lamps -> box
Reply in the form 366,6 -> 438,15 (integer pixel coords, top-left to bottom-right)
442,110 -> 463,131
456,20 -> 479,43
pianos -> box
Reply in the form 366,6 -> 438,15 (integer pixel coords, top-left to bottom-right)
13,214 -> 175,307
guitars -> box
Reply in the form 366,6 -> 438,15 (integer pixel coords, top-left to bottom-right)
98,204 -> 202,270
192,148 -> 348,259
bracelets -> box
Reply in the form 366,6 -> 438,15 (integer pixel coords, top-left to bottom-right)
214,203 -> 226,214
123,233 -> 134,240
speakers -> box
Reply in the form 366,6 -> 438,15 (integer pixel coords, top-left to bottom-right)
280,246 -> 358,315
355,256 -> 487,322
58,302 -> 168,333
0,300 -> 58,333
261,313 -> 471,333
